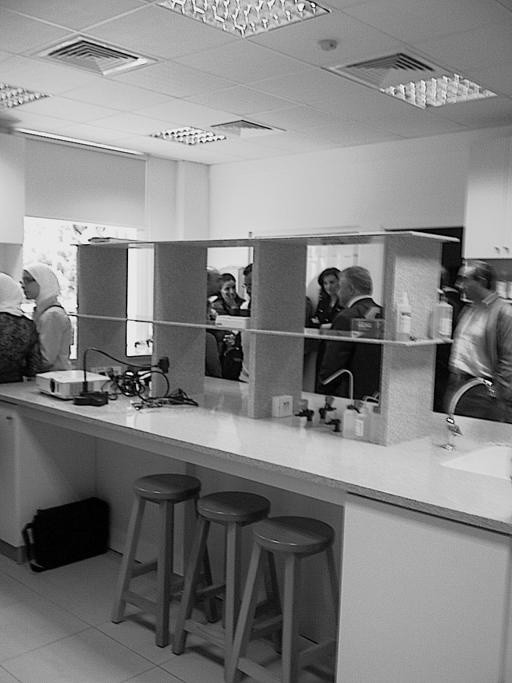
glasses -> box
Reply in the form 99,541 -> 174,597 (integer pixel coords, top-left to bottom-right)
19,276 -> 35,286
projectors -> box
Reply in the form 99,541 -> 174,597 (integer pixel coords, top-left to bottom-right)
36,370 -> 111,399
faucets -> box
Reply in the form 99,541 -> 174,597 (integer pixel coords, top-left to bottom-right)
445,377 -> 498,435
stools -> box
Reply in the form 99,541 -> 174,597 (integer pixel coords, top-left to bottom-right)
171,489 -> 285,670
222,513 -> 337,681
111,470 -> 220,649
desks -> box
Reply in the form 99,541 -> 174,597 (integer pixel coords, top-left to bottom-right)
1,375 -> 512,683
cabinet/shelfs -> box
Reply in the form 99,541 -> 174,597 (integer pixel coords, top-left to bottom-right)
69,229 -> 461,444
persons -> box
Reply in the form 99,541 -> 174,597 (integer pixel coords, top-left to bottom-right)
0,273 -> 42,385
433,259 -> 511,424
20,262 -> 74,372
207,262 -> 252,381
303,266 -> 383,403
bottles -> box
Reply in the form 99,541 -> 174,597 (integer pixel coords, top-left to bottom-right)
341,404 -> 380,442
430,295 -> 452,339
396,295 -> 412,340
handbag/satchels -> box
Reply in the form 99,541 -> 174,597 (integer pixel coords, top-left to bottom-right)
32,496 -> 110,569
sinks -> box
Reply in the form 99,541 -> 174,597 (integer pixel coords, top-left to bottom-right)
442,443 -> 512,480
